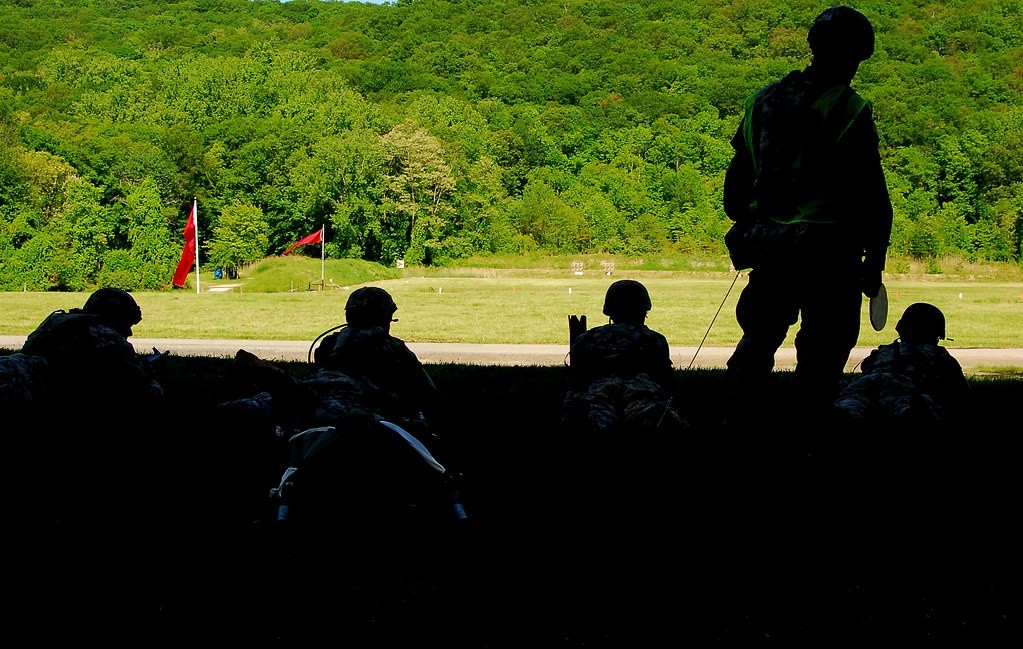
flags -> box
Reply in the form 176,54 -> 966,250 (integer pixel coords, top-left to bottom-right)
173,206 -> 195,287
284,229 -> 323,256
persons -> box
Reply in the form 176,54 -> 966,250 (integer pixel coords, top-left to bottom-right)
831,303 -> 973,448
207,287 -> 438,428
723,7 -> 893,434
571,280 -> 696,453
0,288 -> 172,422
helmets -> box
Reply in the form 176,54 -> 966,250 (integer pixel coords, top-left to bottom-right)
808,6 -> 875,61
346,287 -> 397,329
603,281 -> 651,316
83,288 -> 142,323
896,303 -> 945,342
